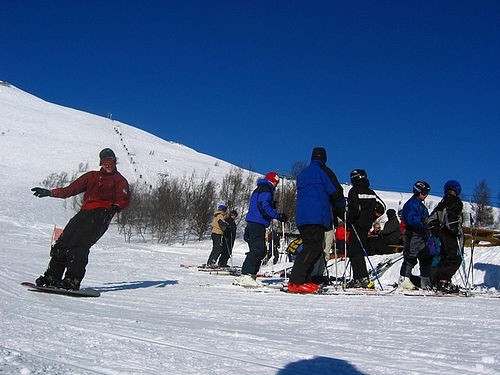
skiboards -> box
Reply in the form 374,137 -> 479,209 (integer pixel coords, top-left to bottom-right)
276,284 -> 398,298
406,287 -> 474,298
231,278 -> 289,289
179,264 -> 243,272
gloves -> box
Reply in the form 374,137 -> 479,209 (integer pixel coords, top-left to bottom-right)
31,187 -> 51,197
273,200 -> 278,208
277,213 -> 288,222
109,203 -> 121,215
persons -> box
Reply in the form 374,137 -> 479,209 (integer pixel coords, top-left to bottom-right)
287,147 -> 345,293
262,226 -> 281,266
399,181 -> 430,291
241,172 -> 279,286
207,204 -> 228,267
372,222 -> 380,233
398,211 -> 405,241
428,180 -> 465,294
32,149 -> 129,289
379,209 -> 400,245
346,169 -> 386,288
218,211 -> 238,267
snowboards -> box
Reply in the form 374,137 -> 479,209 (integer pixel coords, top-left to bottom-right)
21,280 -> 101,298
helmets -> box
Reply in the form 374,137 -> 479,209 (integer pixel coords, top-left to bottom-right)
413,181 -> 431,196
443,180 -> 462,196
350,169 -> 366,184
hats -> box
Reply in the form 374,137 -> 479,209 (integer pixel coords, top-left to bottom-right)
266,172 -> 280,182
99,148 -> 116,162
218,205 -> 227,211
311,147 -> 327,161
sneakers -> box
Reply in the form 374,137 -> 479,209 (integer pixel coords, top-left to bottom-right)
304,280 -> 322,292
399,276 -> 421,292
437,281 -> 460,293
363,280 -> 375,288
239,275 -> 258,287
349,277 -> 362,288
57,276 -> 80,290
288,281 -> 316,293
36,272 -> 61,287
